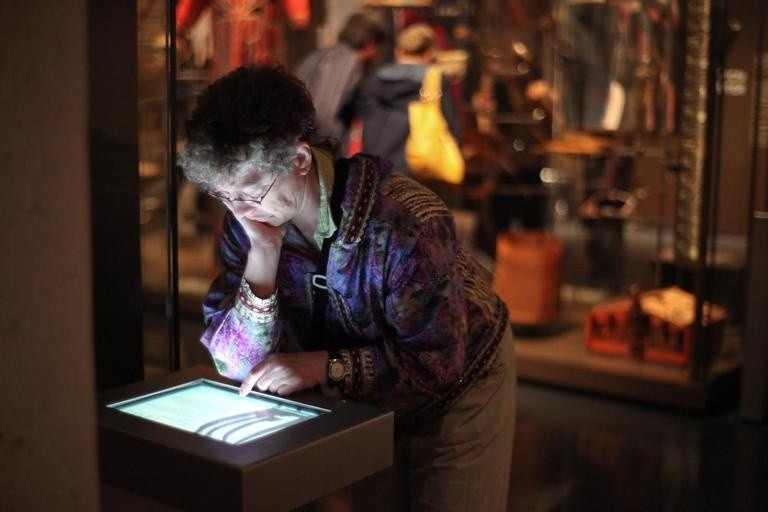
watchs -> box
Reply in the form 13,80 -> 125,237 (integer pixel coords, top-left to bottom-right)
326,349 -> 346,390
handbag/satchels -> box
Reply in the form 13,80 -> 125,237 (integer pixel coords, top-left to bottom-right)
406,100 -> 466,184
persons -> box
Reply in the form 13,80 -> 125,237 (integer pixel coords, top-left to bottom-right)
181,62 -> 518,512
292,14 -> 463,190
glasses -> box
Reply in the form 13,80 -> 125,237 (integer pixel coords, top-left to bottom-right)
207,165 -> 286,208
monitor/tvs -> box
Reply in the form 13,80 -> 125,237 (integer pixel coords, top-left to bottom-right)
106,379 -> 332,445
